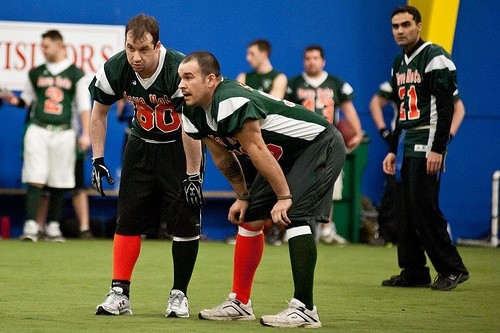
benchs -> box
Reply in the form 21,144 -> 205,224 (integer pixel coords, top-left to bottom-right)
0,189 -> 241,198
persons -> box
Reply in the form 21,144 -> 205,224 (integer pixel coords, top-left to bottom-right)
0,30 -> 92,242
370,79 -> 465,248
228,39 -> 287,245
178,51 -> 346,328
285,45 -> 363,245
382,6 -> 469,290
116,99 -> 150,239
88,14 -> 207,317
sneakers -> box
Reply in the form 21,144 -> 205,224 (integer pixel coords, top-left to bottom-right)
198,292 -> 256,322
260,298 -> 323,329
95,287 -> 132,316
164,287 -> 190,318
381,266 -> 431,288
431,263 -> 469,292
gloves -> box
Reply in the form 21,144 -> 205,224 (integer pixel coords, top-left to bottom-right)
90,156 -> 114,197
184,174 -> 206,207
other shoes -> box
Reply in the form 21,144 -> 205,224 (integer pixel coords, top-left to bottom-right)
225,231 -> 237,245
21,234 -> 39,242
321,232 -> 347,247
46,235 -> 64,243
266,229 -> 279,243
79,231 -> 94,240
368,234 -> 392,249
